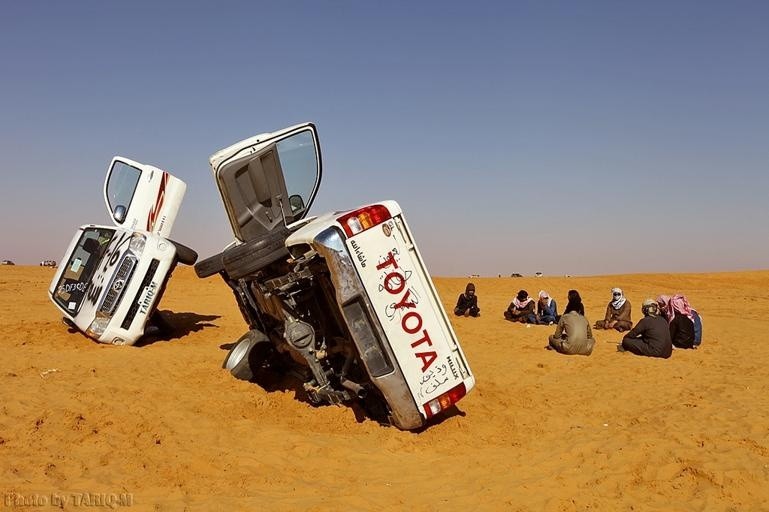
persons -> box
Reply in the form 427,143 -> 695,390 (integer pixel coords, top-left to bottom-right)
504,289 -> 536,324
563,289 -> 584,316
675,297 -> 703,347
653,294 -> 676,324
454,283 -> 481,317
548,302 -> 596,356
616,298 -> 673,360
590,287 -> 634,333
527,289 -> 557,326
667,295 -> 695,349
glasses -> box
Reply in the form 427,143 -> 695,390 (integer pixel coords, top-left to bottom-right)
613,293 -> 621,295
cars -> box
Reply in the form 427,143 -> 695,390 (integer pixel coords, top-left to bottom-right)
47,155 -> 199,347
2,259 -> 15,265
194,120 -> 477,433
40,260 -> 56,266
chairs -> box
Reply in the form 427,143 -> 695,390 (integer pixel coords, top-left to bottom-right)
63,280 -> 84,302
82,238 -> 108,267
